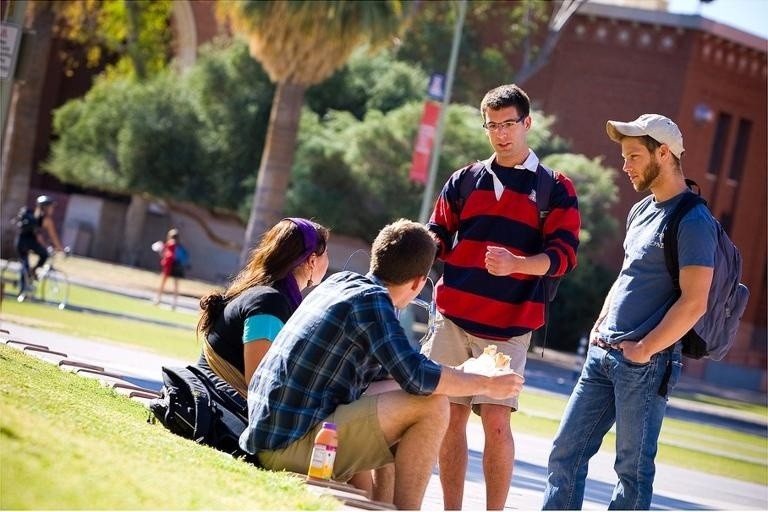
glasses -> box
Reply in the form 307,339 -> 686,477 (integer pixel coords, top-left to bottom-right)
483,116 -> 524,132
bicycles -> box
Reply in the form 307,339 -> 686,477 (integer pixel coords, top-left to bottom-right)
1,244 -> 75,309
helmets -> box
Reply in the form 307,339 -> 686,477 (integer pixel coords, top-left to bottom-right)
36,195 -> 53,205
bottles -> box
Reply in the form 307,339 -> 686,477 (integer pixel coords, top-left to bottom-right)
307,421 -> 337,483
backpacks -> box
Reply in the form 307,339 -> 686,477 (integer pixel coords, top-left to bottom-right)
150,365 -> 258,467
15,207 -> 33,229
664,196 -> 750,362
455,162 -> 561,302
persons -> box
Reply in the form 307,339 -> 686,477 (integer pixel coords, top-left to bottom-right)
196,218 -> 331,510
239,218 -> 525,510
16,195 -> 61,292
425,84 -> 581,511
153,228 -> 186,310
541,113 -> 718,511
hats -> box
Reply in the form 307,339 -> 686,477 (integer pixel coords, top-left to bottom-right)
606,113 -> 686,161
